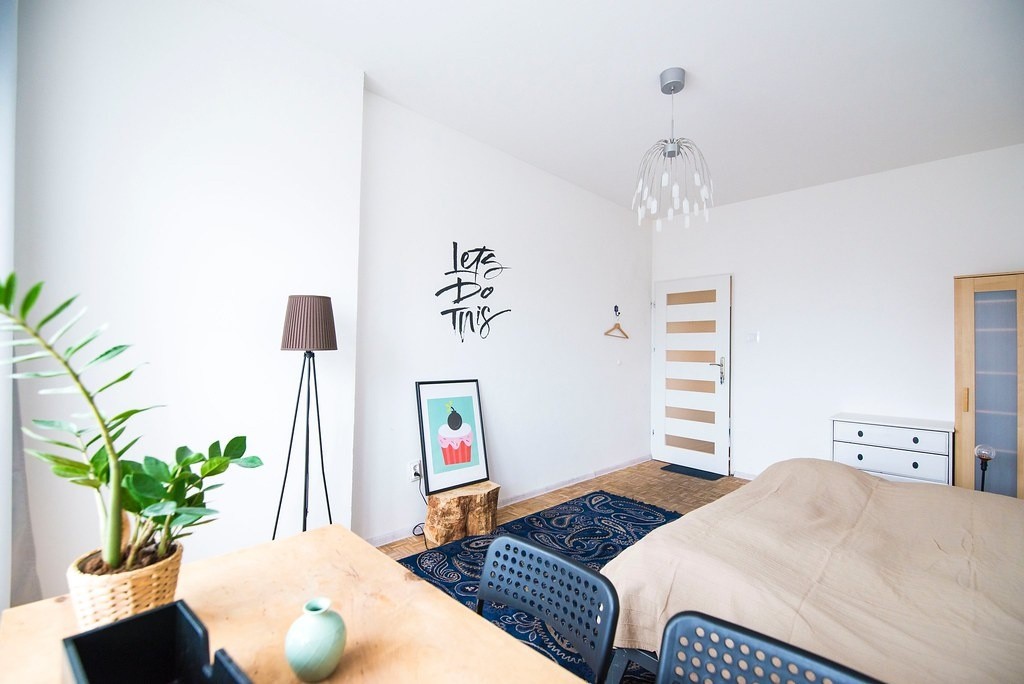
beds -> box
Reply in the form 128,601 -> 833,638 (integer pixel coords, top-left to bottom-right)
602,457 -> 1024,684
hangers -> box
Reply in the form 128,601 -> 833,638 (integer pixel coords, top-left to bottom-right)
604,313 -> 630,340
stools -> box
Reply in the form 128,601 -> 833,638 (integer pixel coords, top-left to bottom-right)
423,480 -> 502,545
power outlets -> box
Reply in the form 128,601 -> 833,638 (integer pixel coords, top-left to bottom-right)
408,459 -> 423,483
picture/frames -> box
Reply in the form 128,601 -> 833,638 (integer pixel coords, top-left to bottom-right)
415,379 -> 490,496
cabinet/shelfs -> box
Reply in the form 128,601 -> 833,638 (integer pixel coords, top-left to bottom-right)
828,412 -> 955,485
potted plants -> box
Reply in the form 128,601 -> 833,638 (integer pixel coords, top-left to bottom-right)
0,273 -> 262,633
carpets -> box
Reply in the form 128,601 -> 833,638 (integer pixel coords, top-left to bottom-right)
396,490 -> 685,684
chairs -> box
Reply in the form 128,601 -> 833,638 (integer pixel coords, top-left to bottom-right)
474,535 -> 886,684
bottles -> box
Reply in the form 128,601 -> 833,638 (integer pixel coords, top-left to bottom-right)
284,597 -> 346,682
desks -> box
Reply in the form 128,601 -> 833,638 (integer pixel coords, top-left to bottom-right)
0,522 -> 600,684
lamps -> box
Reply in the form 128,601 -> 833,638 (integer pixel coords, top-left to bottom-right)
273,294 -> 343,543
630,69 -> 723,232
